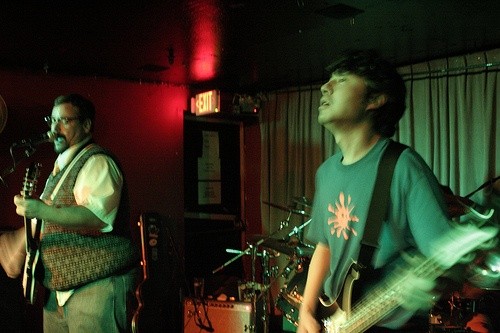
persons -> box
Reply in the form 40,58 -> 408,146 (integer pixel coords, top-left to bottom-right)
295,47 -> 476,333
1,93 -> 139,333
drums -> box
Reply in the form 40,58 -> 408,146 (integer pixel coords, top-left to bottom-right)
456,253 -> 500,299
279,257 -> 310,309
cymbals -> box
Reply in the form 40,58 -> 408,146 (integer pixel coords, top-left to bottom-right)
296,196 -> 312,207
246,233 -> 315,256
262,201 -> 309,218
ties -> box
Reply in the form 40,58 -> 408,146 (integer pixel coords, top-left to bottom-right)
53,162 -> 59,176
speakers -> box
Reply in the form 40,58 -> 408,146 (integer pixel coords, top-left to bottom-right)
136,210 -> 165,287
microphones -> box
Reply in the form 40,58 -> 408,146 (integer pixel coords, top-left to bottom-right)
12,131 -> 55,147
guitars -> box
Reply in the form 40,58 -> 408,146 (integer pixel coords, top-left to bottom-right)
21,162 -> 42,305
315,226 -> 498,333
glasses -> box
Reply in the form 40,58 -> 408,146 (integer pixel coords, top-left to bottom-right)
44,116 -> 79,124
